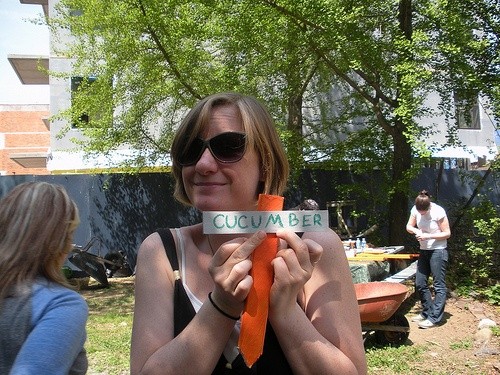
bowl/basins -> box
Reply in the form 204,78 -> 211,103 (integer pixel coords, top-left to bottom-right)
354,281 -> 409,322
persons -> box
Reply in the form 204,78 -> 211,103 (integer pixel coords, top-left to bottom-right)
130,93 -> 368,375
0,181 -> 88,375
289,199 -> 319,237
406,192 -> 451,328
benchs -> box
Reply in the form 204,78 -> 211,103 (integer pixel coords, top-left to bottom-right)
382,261 -> 417,283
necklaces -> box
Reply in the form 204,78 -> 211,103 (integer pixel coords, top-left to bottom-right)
208,235 -> 214,255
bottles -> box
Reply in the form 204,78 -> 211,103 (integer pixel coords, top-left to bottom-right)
362,238 -> 366,249
356,238 -> 361,249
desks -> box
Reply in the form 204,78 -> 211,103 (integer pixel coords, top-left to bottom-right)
345,245 -> 406,284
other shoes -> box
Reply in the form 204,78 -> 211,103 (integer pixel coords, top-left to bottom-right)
411,314 -> 424,322
418,318 -> 438,329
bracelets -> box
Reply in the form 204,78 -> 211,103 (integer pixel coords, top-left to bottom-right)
208,291 -> 241,321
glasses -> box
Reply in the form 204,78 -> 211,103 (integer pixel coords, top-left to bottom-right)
172,131 -> 247,168
